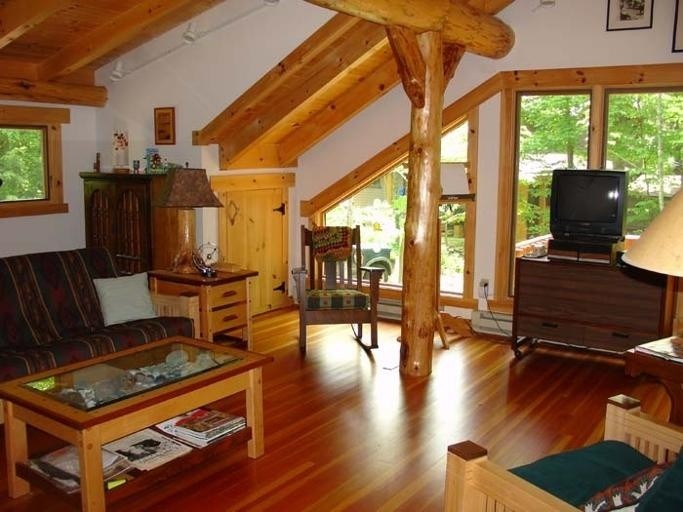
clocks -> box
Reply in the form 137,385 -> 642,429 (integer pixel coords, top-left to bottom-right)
191,242 -> 222,277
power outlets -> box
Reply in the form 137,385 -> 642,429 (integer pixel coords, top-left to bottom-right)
479,278 -> 488,287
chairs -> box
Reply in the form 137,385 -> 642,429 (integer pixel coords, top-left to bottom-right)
441,391 -> 682,512
291,223 -> 386,360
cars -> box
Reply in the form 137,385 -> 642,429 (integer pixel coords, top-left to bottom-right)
336,240 -> 396,282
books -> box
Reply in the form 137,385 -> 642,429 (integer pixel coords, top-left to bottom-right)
25,405 -> 246,497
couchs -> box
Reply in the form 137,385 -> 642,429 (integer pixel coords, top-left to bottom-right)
0,240 -> 196,427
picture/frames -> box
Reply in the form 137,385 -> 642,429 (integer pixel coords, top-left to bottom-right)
670,0 -> 682,53
152,107 -> 176,145
604,0 -> 654,32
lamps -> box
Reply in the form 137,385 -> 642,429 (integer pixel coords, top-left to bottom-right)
619,187 -> 683,351
94,0 -> 282,89
154,161 -> 224,275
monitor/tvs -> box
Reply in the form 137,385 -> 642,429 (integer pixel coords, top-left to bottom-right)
550,169 -> 629,241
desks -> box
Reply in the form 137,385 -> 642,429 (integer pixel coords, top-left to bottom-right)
623,335 -> 682,425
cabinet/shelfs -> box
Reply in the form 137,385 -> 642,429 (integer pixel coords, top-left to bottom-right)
77,171 -> 179,291
146,262 -> 259,350
509,255 -> 674,361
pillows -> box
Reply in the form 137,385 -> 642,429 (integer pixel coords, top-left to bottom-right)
92,271 -> 157,327
